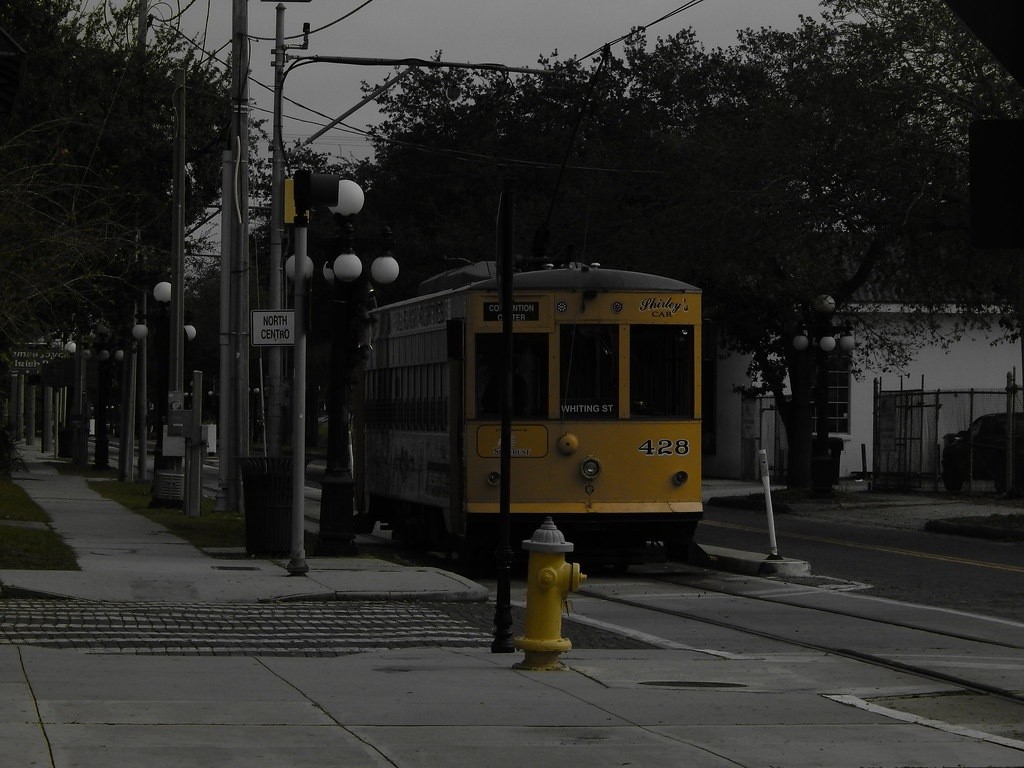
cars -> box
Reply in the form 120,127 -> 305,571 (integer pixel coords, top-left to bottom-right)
944,408 -> 1023,500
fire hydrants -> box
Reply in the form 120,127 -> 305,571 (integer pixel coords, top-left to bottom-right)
520,515 -> 589,671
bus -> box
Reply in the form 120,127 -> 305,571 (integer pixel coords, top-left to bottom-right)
357,257 -> 707,555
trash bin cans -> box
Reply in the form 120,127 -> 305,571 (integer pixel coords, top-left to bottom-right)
231,454 -> 321,558
813,437 -> 844,484
153,469 -> 187,500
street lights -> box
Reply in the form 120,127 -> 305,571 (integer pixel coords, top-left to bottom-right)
91,318 -> 125,474
283,177 -> 402,561
796,300 -> 856,460
133,282 -> 198,482
